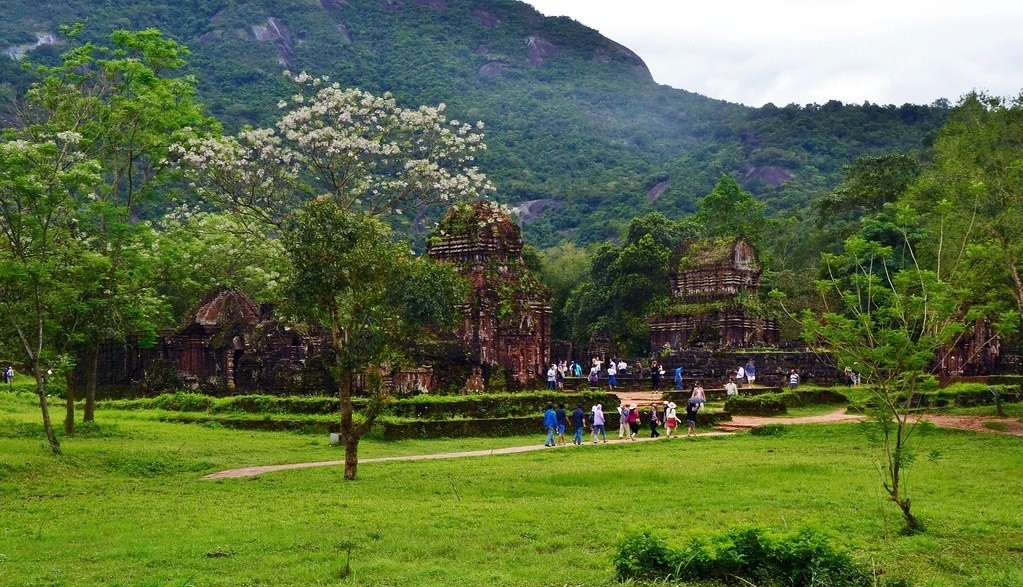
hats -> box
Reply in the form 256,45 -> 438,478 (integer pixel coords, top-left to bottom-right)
620,403 -> 625,409
662,401 -> 669,404
853,362 -> 859,365
651,404 -> 657,409
591,406 -> 596,411
628,404 -> 637,410
668,402 -> 676,408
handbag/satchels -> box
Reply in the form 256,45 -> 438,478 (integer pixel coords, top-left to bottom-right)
636,419 -> 641,426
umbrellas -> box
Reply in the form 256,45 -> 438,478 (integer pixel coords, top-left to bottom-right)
688,397 -> 705,405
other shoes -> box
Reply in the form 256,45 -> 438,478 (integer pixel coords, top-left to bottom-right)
656,433 -> 659,437
631,435 -> 635,441
594,441 -> 599,443
674,436 -> 678,438
604,440 -> 608,443
552,444 -> 555,446
573,439 -> 576,445
666,435 -> 669,439
545,444 -> 551,447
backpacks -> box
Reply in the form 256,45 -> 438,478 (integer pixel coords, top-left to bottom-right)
587,413 -> 594,423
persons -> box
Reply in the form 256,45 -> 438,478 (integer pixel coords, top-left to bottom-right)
674,365 -> 683,390
590,404 -> 608,443
644,404 -> 661,438
845,362 -> 860,388
734,365 -> 744,388
2,367 -> 14,383
691,380 -> 705,409
650,361 -> 662,394
589,356 -> 605,387
723,378 -> 738,399
686,403 -> 700,437
557,359 -> 582,388
660,401 -> 681,438
619,403 -> 640,441
573,403 -> 587,445
555,402 -> 571,446
745,359 -> 755,388
790,369 -> 799,390
543,401 -> 559,447
547,365 -> 557,390
607,357 -> 627,390
